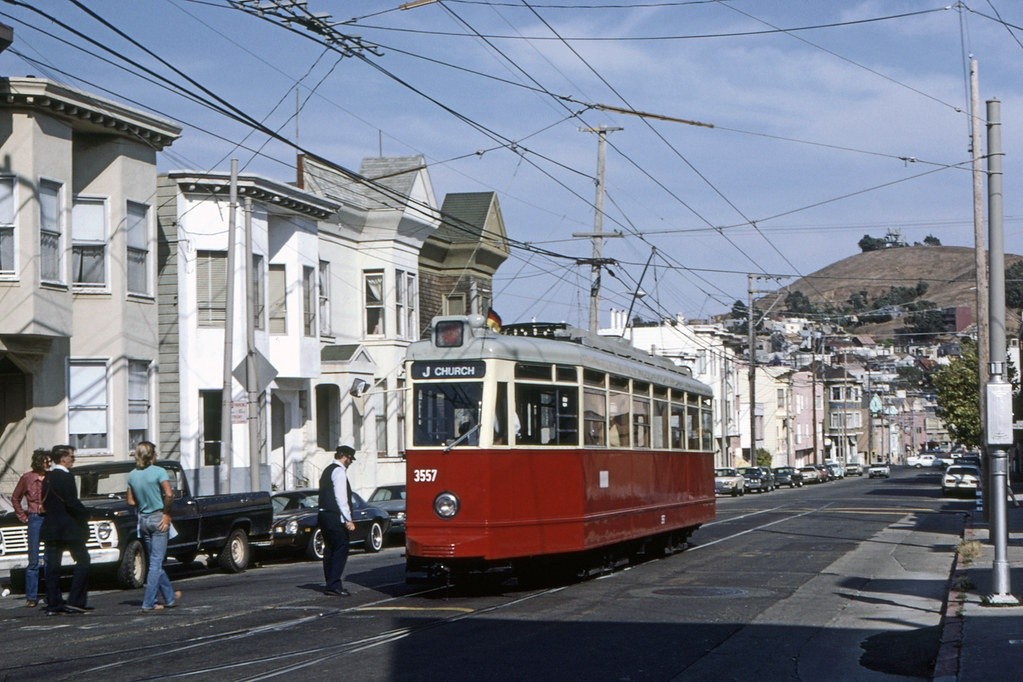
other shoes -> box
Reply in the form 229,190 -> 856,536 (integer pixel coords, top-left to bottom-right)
167,590 -> 181,609
143,600 -> 166,610
64,602 -> 93,612
28,599 -> 36,607
48,607 -> 66,616
324,588 -> 350,597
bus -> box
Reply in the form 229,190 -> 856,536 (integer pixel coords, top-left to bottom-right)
400,246 -> 718,589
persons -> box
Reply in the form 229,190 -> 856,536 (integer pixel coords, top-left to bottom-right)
317,445 -> 357,598
591,400 -> 623,447
493,382 -> 522,446
11,448 -> 51,609
128,441 -> 183,613
38,445 -> 93,616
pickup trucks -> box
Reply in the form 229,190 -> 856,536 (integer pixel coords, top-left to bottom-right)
906,454 -> 955,470
0,460 -> 274,594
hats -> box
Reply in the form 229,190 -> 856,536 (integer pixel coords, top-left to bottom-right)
336,446 -> 356,461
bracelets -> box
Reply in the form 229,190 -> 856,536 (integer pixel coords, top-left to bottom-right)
164,505 -> 171,513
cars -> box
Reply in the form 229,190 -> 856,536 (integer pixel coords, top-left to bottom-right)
249,487 -> 391,561
743,462 -> 843,493
868,462 -> 891,478
942,456 -> 981,495
713,467 -> 746,498
366,484 -> 406,535
844,462 -> 863,477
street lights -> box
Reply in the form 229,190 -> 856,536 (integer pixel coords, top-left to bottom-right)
588,288 -> 648,333
